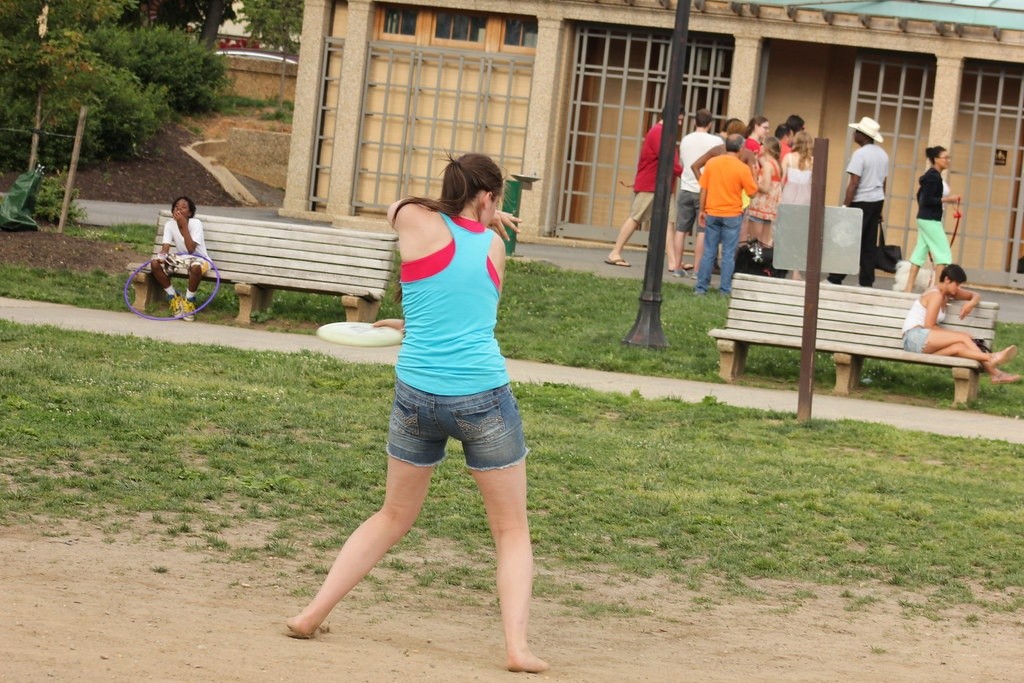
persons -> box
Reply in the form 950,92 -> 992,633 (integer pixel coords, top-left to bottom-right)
782,131 -> 814,281
287,154 -> 551,673
151,196 -> 213,322
903,146 -> 960,293
691,115 -> 805,278
692,133 -> 759,297
819,116 -> 889,287
902,264 -> 1021,383
604,105 -> 694,272
672,109 -> 723,280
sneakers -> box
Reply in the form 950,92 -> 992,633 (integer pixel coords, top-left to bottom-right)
168,289 -> 184,317
182,294 -> 198,322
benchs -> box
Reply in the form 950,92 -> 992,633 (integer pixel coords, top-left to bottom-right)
126,210 -> 400,326
708,272 -> 1001,404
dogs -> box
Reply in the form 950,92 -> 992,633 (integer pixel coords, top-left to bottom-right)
892,261 -> 935,292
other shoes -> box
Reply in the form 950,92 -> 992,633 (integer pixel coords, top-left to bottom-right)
989,344 -> 1018,368
820,278 -> 832,285
691,271 -> 699,281
672,268 -> 689,278
991,372 -> 1021,383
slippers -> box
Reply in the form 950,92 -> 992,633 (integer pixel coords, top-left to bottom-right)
667,263 -> 694,272
604,258 -> 632,267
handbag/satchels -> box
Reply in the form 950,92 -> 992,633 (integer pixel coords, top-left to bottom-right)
874,223 -> 902,273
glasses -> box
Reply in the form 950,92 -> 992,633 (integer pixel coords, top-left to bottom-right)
760,125 -> 769,130
759,142 -> 763,146
799,127 -> 805,130
937,156 -> 951,162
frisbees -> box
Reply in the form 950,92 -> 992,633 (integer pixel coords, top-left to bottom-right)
314,320 -> 404,349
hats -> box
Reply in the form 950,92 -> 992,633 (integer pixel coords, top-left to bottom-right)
848,115 -> 884,143
